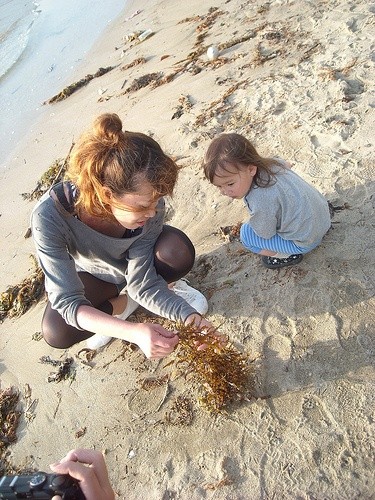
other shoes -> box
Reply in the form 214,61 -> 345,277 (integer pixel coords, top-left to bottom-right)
262,254 -> 303,269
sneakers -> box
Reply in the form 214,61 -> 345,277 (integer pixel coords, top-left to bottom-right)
87,295 -> 140,348
171,280 -> 208,315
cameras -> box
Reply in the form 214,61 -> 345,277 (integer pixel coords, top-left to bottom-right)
0,471 -> 86,500
